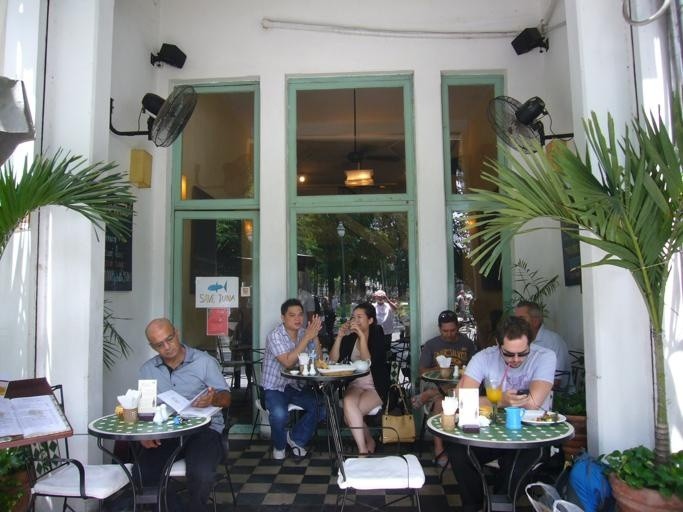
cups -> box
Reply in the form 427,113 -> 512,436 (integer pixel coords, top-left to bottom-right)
504,407 -> 527,430
447,388 -> 459,400
123,407 -> 138,424
300,364 -> 309,373
440,368 -> 452,379
441,413 -> 458,435
344,325 -> 355,336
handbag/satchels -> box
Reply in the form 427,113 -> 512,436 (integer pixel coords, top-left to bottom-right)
569,453 -> 613,511
381,383 -> 418,444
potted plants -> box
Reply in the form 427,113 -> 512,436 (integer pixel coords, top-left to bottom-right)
452,102 -> 682,512
0,146 -> 138,512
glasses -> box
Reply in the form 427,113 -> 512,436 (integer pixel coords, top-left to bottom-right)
439,312 -> 456,318
499,345 -> 531,357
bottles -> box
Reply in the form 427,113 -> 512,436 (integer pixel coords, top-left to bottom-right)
160,404 -> 170,423
453,365 -> 460,379
302,364 -> 310,377
152,407 -> 163,424
309,364 -> 317,375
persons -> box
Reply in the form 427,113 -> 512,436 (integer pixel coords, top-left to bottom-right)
132,318 -> 231,512
411,311 -> 475,469
259,299 -> 323,460
329,303 -> 390,459
516,302 -> 577,394
228,296 -> 252,391
449,317 -> 557,512
298,271 -> 473,348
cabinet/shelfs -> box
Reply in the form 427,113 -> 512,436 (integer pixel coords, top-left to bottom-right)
188,185 -> 243,297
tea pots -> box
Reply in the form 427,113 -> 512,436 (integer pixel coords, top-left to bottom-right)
348,359 -> 373,372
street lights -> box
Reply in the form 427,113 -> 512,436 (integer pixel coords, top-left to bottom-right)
335,218 -> 347,324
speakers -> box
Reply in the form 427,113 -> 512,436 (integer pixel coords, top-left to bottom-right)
158,43 -> 186,69
511,27 -> 543,55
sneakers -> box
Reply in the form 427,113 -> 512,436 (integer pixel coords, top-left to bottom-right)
272,431 -> 307,459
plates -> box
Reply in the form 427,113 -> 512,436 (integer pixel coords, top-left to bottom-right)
291,371 -> 299,375
319,369 -> 353,376
519,409 -> 567,426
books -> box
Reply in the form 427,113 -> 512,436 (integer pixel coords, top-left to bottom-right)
156,388 -> 223,418
0,395 -> 71,439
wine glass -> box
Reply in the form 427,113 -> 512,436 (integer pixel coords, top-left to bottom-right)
485,378 -> 503,426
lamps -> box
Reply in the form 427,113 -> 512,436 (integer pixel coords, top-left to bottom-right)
150,40 -> 186,70
342,88 -> 376,188
337,218 -> 345,238
508,27 -> 549,58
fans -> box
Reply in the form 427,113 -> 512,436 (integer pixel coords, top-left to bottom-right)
109,82 -> 199,149
486,94 -> 574,154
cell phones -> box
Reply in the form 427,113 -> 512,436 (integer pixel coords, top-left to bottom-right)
516,389 -> 529,395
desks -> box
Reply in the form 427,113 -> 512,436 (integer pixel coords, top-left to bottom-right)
87,410 -> 212,511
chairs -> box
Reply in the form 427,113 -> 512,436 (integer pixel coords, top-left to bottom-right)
217,340 -> 571,500
127,408 -> 239,510
426,410 -> 577,511
23,385 -> 138,512
325,397 -> 421,512
